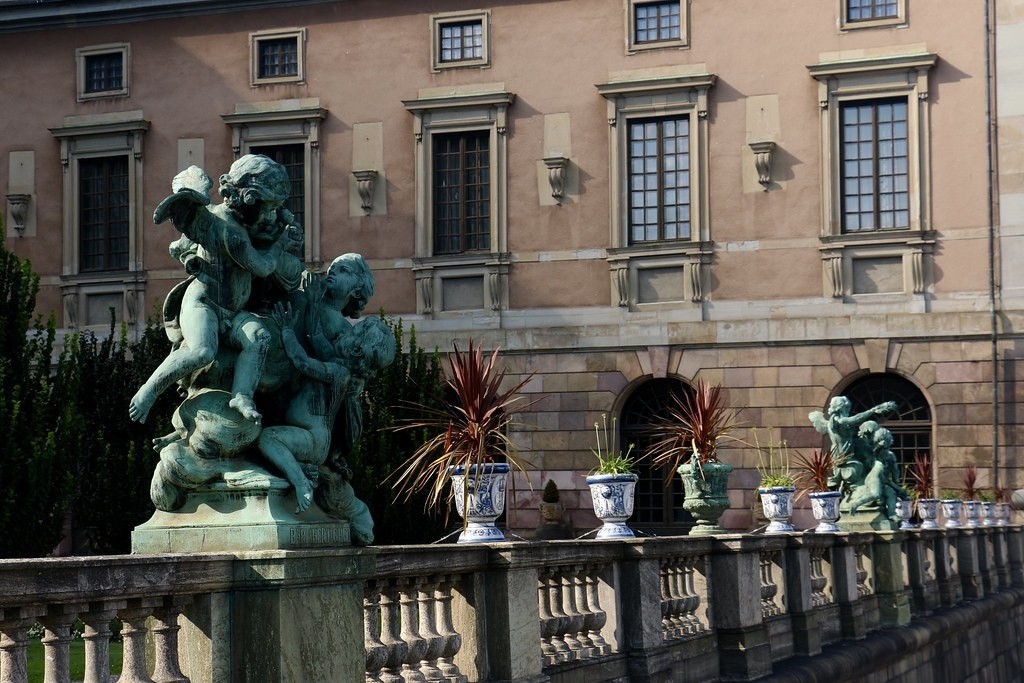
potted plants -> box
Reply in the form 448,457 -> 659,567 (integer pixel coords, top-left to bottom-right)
537,479 -> 566,525
583,414 -> 639,537
376,337 -> 551,541
751,425 -> 795,533
895,449 -> 1024,529
636,377 -> 771,537
791,440 -> 854,534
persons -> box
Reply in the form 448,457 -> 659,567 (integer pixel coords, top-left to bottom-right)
806,395 -> 906,522
126,154 -> 398,546
